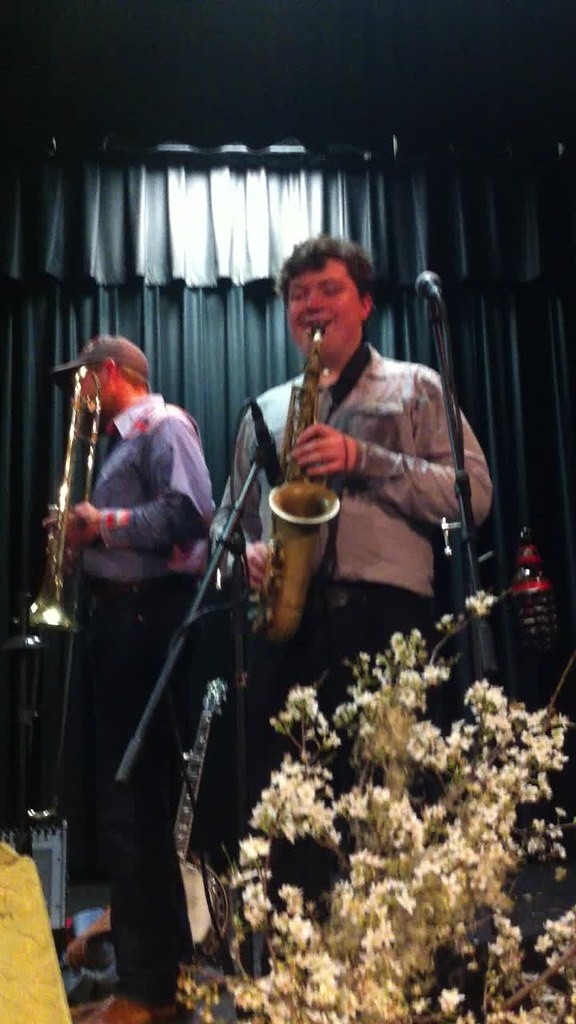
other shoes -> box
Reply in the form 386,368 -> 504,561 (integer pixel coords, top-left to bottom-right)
81,994 -> 184,1024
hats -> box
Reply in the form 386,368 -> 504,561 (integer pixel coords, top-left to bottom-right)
50,337 -> 149,393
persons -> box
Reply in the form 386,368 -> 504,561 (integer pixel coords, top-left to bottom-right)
210,238 -> 492,1022
48,336 -> 217,1024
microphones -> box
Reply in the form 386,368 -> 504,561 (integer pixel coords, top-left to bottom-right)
416,270 -> 443,298
250,400 -> 284,487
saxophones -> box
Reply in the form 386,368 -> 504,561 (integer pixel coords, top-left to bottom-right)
260,328 -> 343,633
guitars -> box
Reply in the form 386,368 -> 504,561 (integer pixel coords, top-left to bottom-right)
174,676 -> 229,951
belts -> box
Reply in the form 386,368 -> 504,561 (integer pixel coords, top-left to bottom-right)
96,582 -> 194,595
313,581 -> 422,607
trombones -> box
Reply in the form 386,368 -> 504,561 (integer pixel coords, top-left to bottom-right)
9,363 -> 105,822
